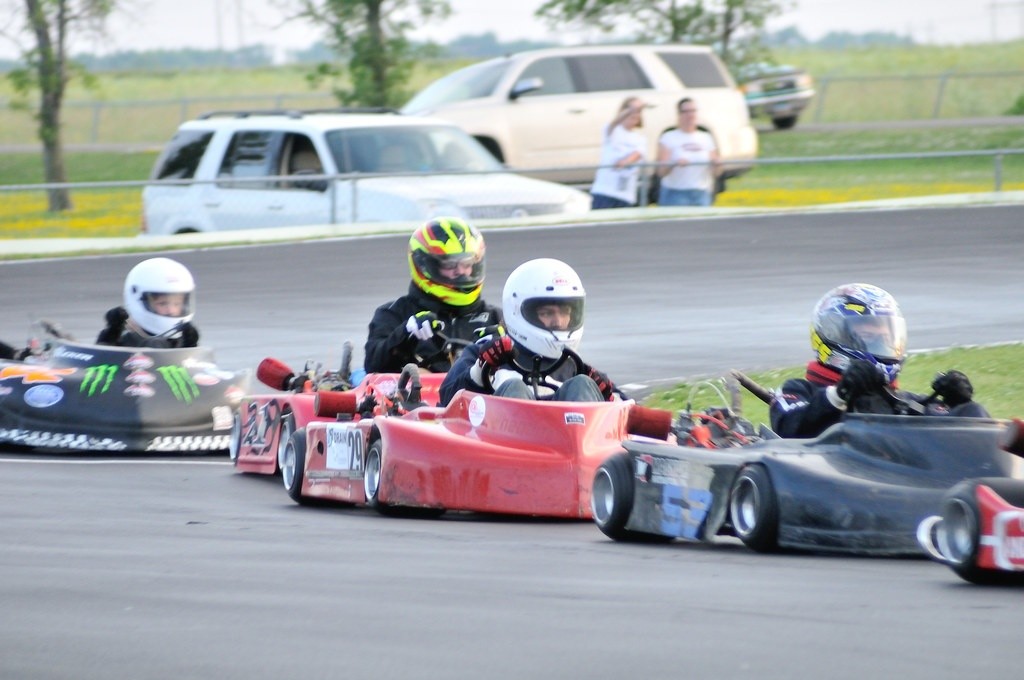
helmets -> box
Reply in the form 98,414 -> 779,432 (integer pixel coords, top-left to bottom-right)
123,257 -> 196,337
808,282 -> 907,384
407,217 -> 486,307
502,258 -> 586,359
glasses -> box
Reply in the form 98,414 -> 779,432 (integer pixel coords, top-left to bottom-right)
436,259 -> 474,270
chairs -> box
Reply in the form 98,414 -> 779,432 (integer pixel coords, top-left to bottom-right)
380,146 -> 412,172
290,153 -> 321,170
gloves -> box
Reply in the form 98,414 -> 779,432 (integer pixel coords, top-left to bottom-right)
932,370 -> 974,407
479,336 -> 516,372
405,310 -> 438,341
582,362 -> 614,403
836,359 -> 884,403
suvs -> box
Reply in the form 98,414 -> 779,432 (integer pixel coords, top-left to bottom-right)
143,109 -> 593,235
386,44 -> 758,191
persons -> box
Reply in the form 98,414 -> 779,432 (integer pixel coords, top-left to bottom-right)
364,216 -> 504,373
770,283 -> 973,439
93,259 -> 202,348
441,260 -> 627,410
592,90 -> 655,204
649,95 -> 724,206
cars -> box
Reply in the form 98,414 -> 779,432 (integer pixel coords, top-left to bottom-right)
730,62 -> 816,130
